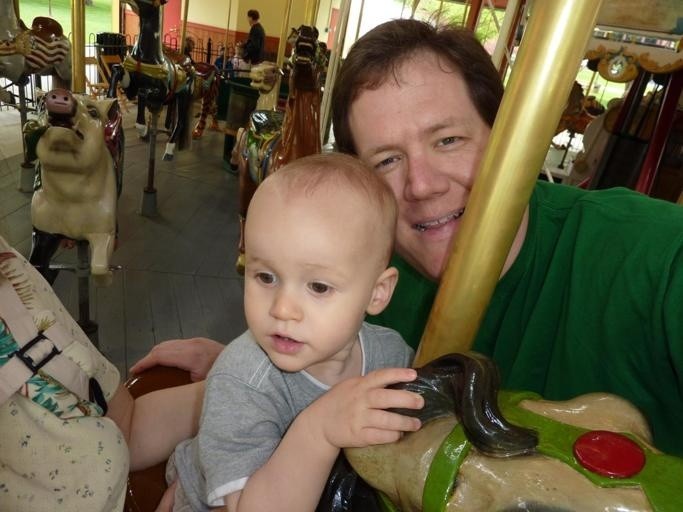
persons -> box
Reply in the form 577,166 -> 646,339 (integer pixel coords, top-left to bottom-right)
159,146 -> 428,512
124,15 -> 682,464
236,9 -> 266,65
0,238 -> 211,511
212,46 -> 235,82
183,34 -> 195,61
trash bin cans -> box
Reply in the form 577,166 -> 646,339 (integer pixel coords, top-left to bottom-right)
224,77 -> 258,167
